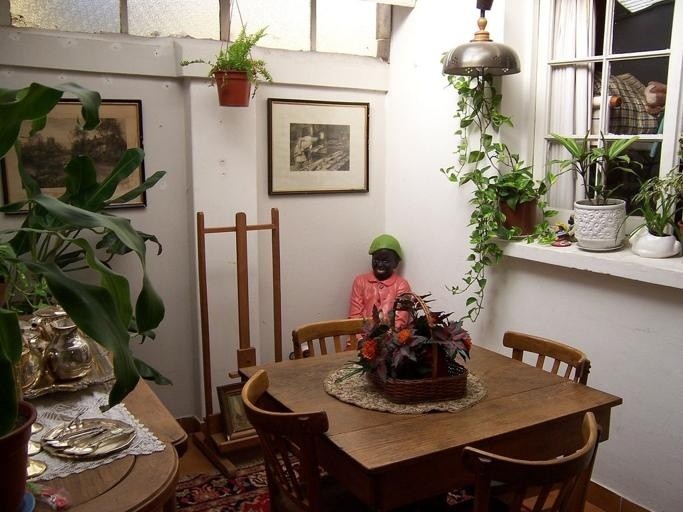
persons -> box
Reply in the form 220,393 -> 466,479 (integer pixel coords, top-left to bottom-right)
294,133 -> 321,171
347,234 -> 411,348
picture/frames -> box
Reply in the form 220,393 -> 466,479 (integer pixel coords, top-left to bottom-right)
1,99 -> 147,216
217,383 -> 257,439
267,98 -> 369,196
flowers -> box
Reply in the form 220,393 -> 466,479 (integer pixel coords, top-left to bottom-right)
343,293 -> 472,385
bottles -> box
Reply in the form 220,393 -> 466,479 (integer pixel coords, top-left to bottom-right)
45,319 -> 92,381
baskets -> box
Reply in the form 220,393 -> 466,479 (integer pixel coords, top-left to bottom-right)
366,292 -> 469,404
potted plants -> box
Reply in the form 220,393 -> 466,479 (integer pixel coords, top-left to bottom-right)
666,133 -> 683,236
1,82 -> 173,511
491,160 -> 559,246
545,129 -> 644,251
178,21 -> 272,107
615,177 -> 682,258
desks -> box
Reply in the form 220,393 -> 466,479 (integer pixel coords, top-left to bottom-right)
239,343 -> 622,512
25,342 -> 189,511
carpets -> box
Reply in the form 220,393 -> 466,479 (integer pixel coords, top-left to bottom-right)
167,452 -> 532,511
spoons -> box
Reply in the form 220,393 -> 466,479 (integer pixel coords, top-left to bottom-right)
44,427 -> 60,441
52,431 -> 94,447
47,427 -> 102,445
64,427 -> 124,453
79,428 -> 134,454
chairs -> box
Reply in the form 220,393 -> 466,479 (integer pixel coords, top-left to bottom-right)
291,318 -> 374,358
240,369 -> 370,512
450,412 -> 603,512
503,331 -> 591,386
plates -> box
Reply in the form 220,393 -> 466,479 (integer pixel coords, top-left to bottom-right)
41,418 -> 137,458
